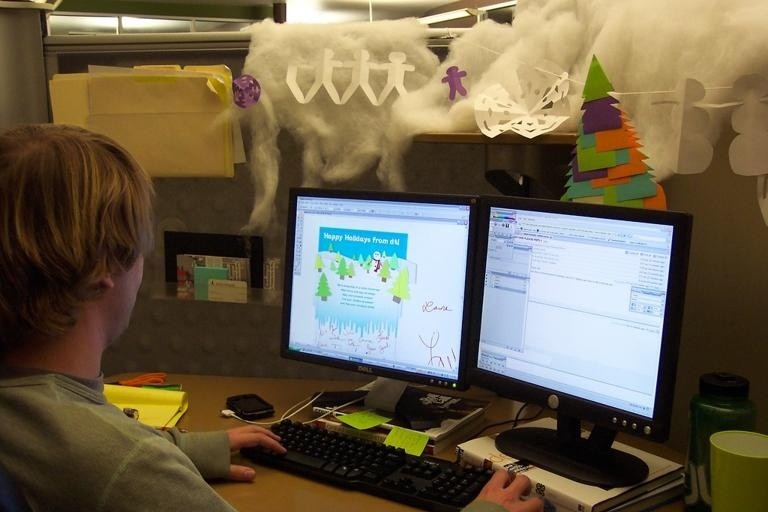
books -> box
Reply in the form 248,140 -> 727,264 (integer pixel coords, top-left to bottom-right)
312,378 -> 494,456
455,414 -> 687,510
177,251 -> 284,303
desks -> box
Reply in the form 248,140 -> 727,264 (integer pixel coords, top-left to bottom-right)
101,372 -> 688,512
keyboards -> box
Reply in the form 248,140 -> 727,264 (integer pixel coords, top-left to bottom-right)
241,418 -> 495,510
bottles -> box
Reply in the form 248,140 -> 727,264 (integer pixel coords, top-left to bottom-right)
686,374 -> 753,511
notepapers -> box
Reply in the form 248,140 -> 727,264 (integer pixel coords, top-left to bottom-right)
338,408 -> 391,430
383,426 -> 430,457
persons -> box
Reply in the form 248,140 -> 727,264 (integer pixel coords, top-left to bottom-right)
0,124 -> 547,512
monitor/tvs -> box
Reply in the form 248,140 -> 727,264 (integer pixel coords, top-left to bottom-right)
467,190 -> 693,488
281,188 -> 479,412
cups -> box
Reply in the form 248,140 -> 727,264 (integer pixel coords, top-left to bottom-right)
709,430 -> 768,512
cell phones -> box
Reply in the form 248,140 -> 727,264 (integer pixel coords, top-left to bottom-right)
226,393 -> 275,421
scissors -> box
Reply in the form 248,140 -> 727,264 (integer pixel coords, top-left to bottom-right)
104,371 -> 167,387
